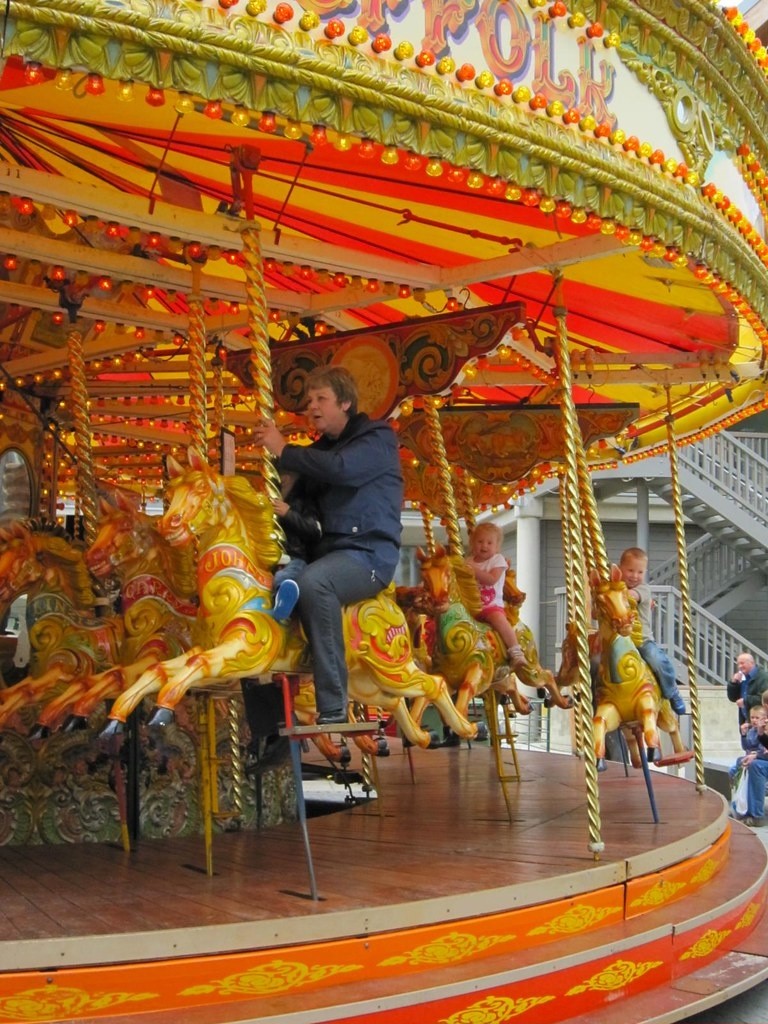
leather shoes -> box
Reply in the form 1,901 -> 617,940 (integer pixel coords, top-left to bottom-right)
316,704 -> 348,724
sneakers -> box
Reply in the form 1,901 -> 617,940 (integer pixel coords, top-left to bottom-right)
670,693 -> 686,714
507,648 -> 529,667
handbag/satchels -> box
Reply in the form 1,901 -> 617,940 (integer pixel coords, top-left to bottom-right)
731,766 -> 750,818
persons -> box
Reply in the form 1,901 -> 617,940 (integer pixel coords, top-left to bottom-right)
727,653 -> 768,827
238,460 -> 321,767
464,523 -> 528,668
252,365 -> 402,725
592,548 -> 686,715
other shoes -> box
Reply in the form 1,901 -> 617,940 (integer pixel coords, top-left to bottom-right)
273,578 -> 300,621
736,814 -> 764,827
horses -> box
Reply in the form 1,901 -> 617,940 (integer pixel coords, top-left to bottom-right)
0,448 -> 686,776
